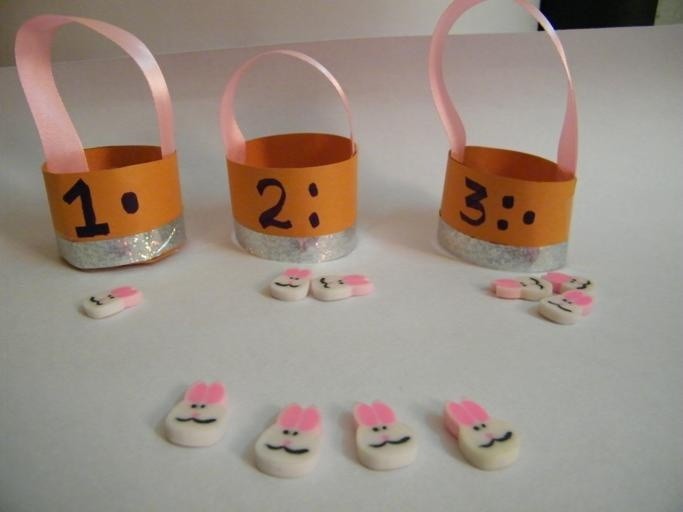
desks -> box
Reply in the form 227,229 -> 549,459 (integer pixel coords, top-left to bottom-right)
0,24 -> 683,512
